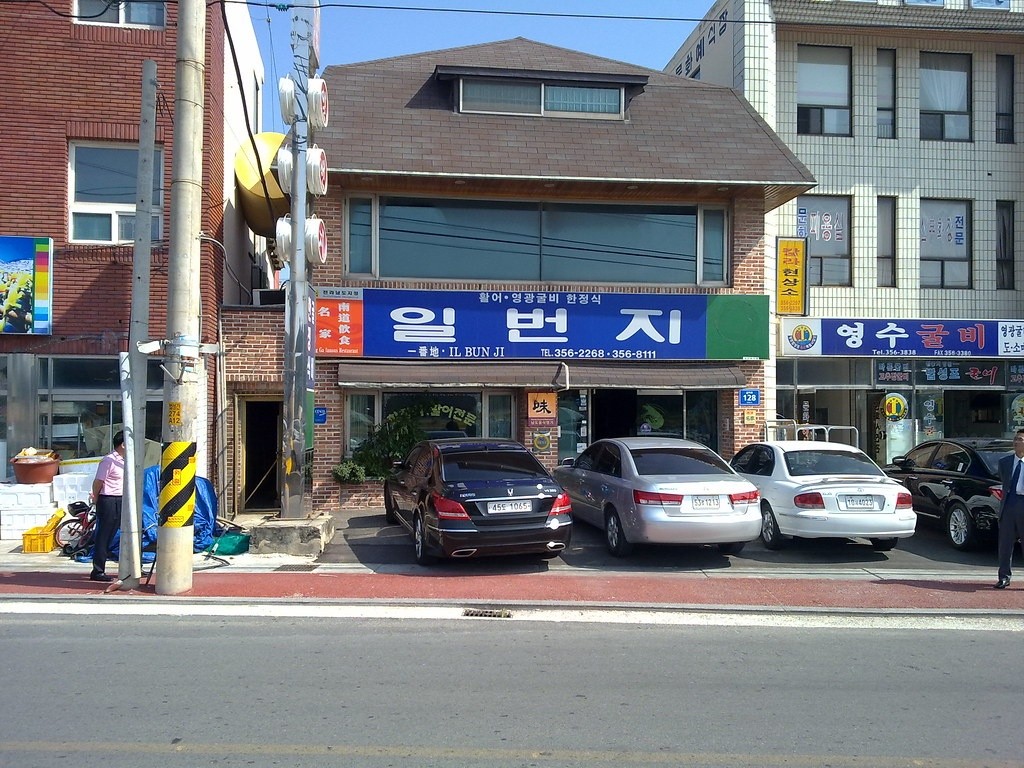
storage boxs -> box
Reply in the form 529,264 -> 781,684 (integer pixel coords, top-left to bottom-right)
22,454 -> 106,555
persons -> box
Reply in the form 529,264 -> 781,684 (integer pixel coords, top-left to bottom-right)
88,428 -> 126,583
994,427 -> 1024,589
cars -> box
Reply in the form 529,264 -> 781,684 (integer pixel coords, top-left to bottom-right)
881,436 -> 1021,551
382,438 -> 573,566
557,428 -> 586,464
725,440 -> 917,551
551,437 -> 764,557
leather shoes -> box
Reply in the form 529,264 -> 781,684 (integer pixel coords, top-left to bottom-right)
91,573 -> 113,581
995,579 -> 1010,588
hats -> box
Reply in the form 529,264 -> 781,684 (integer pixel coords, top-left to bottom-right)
1008,460 -> 1023,508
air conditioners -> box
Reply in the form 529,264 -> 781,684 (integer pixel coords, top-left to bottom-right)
252,288 -> 287,306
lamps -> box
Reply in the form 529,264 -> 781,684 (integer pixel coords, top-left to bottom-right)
136,339 -> 164,354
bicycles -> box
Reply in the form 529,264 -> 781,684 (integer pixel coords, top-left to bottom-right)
53,493 -> 99,551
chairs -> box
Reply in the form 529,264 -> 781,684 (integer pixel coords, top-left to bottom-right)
816,451 -> 844,473
758,457 -> 775,477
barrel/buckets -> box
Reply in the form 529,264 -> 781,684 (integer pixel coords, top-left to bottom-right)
234,131 -> 292,237
